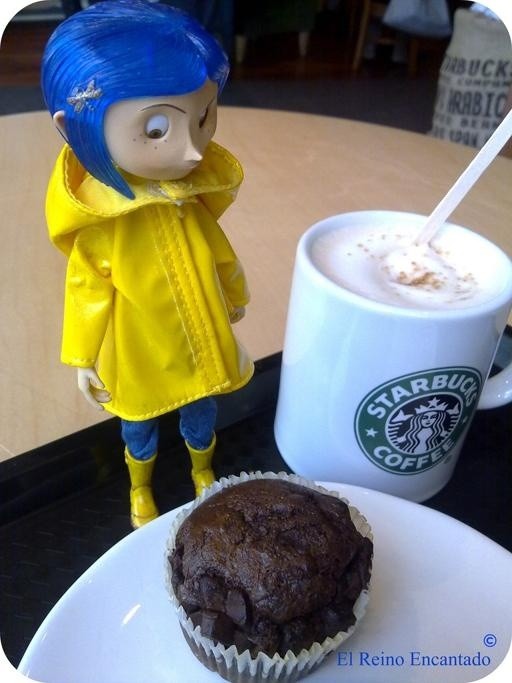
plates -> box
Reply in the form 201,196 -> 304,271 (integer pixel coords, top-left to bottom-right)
17,479 -> 511,683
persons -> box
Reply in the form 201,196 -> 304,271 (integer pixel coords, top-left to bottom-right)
35,2 -> 254,538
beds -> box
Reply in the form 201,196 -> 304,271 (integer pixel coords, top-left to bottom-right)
0,102 -> 510,681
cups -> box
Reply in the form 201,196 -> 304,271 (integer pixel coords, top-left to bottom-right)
274,209 -> 512,503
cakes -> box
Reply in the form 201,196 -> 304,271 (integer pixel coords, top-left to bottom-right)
170,480 -> 374,681
